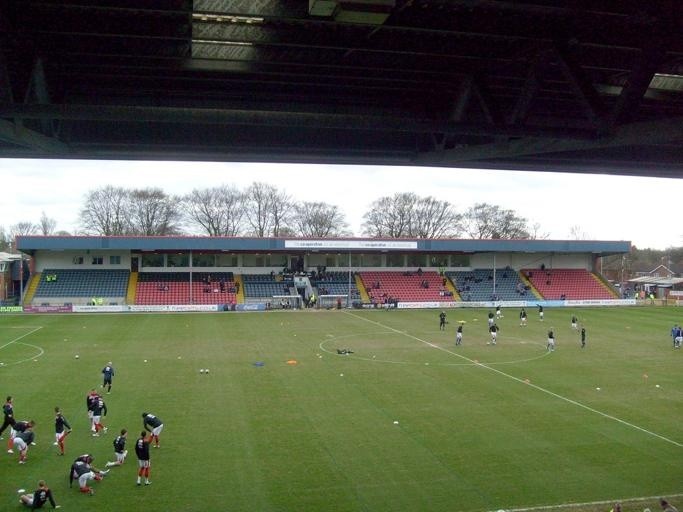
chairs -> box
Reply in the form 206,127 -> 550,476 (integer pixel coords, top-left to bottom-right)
360,270 -> 457,302
31,268 -> 132,304
306,271 -> 363,304
445,269 -> 538,299
241,274 -> 299,306
135,269 -> 238,305
520,269 -> 613,299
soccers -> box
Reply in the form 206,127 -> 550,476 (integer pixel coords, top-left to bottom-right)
596,386 -> 600,390
486,342 -> 490,345
18,489 -> 25,493
654,384 -> 660,388
393,420 -> 398,424
198,369 -> 210,374
75,355 -> 78,359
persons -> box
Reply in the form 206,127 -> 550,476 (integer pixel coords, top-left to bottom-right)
610,499 -> 679,512
540,263 -> 566,300
352,287 -> 363,309
488,313 -> 499,344
101,362 -> 114,394
366,282 -> 397,309
203,274 -> 240,295
571,314 -> 577,327
18,480 -> 61,512
536,305 -> 543,321
458,270 -> 532,301
520,308 -> 527,327
10,389 -> 163,496
0,396 -> 16,440
337,296 -> 341,309
670,324 -> 682,352
439,310 -> 448,331
271,264 -> 333,309
403,267 -> 453,296
624,286 -> 655,306
46,270 -> 57,282
546,327 -> 554,352
580,328 -> 585,348
496,305 -> 502,319
456,322 -> 464,345
87,296 -> 104,306
